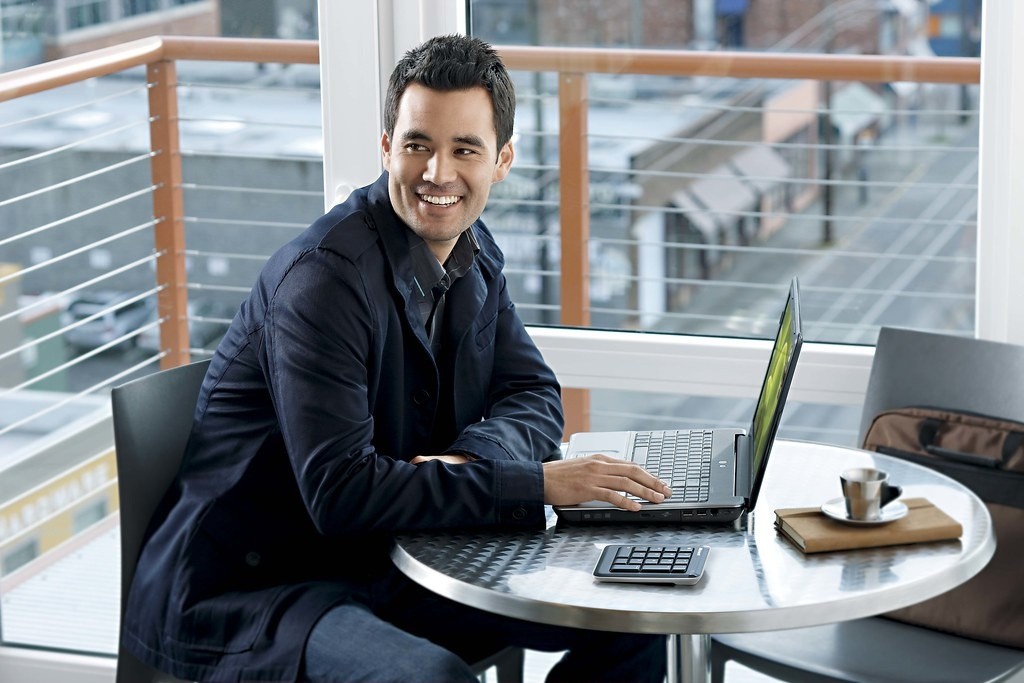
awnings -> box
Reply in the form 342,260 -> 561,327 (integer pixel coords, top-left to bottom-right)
679,82 -> 885,236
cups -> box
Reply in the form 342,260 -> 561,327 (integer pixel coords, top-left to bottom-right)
840,470 -> 901,522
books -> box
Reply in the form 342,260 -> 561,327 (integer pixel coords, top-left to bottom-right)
772,497 -> 962,553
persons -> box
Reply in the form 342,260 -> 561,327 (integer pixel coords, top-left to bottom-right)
117,37 -> 671,683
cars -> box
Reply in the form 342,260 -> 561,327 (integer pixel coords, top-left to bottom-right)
60,289 -> 153,354
135,291 -> 240,352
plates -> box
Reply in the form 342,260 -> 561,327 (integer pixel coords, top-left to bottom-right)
822,495 -> 909,524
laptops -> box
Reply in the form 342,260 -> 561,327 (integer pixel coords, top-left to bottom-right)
552,276 -> 802,524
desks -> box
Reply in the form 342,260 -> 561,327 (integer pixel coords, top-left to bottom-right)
390,439 -> 996,683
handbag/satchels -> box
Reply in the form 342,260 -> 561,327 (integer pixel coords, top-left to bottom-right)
862,408 -> 1024,652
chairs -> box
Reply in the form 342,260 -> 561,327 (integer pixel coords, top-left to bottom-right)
111,358 -> 525,683
710,326 -> 1024,683
